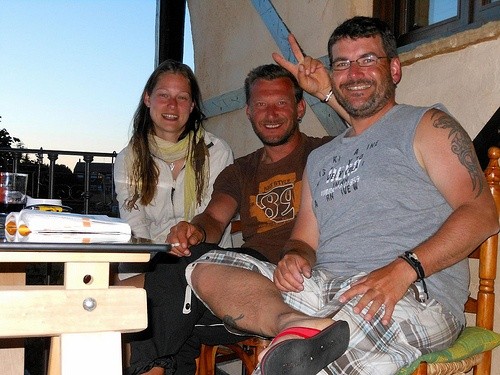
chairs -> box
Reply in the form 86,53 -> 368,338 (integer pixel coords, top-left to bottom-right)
194,146 -> 500,375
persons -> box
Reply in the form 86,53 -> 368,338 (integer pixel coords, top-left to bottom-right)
114,59 -> 237,254
119,32 -> 352,375
185,15 -> 500,375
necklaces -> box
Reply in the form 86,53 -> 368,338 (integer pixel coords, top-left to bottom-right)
169,162 -> 174,172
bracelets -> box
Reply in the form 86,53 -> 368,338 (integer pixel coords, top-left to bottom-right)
197,224 -> 206,244
321,90 -> 334,103
398,251 -> 425,281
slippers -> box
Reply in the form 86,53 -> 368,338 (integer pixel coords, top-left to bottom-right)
260,320 -> 350,375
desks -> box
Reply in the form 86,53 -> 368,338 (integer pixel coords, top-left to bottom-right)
0,230 -> 172,375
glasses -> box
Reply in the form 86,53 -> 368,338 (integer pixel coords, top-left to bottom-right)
329,54 -> 392,70
